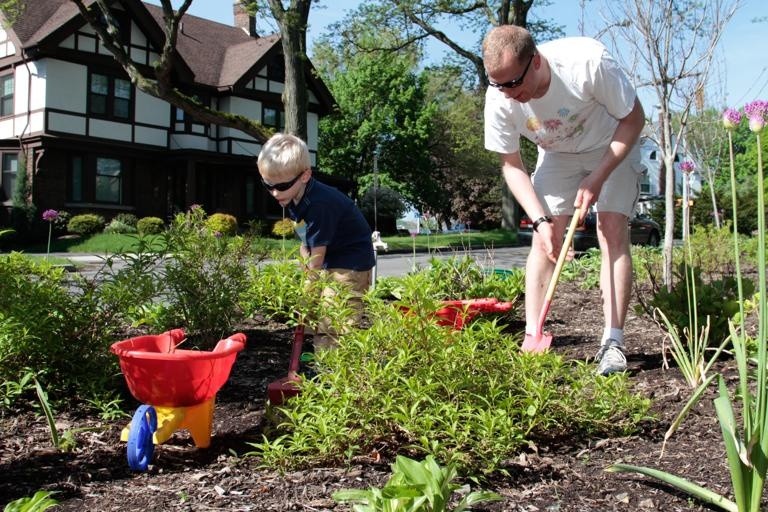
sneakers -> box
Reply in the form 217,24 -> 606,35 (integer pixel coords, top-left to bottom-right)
594,339 -> 628,377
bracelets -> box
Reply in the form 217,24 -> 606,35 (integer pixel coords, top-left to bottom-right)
532,215 -> 552,233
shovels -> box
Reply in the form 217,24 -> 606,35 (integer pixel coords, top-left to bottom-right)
267,326 -> 312,407
519,208 -> 578,357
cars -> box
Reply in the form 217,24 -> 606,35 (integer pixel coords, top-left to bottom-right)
517,213 -> 662,258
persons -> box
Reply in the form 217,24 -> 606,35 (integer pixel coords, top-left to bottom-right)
481,24 -> 647,374
256,133 -> 377,374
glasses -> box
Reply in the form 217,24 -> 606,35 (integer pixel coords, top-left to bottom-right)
261,166 -> 307,192
484,55 -> 533,89
